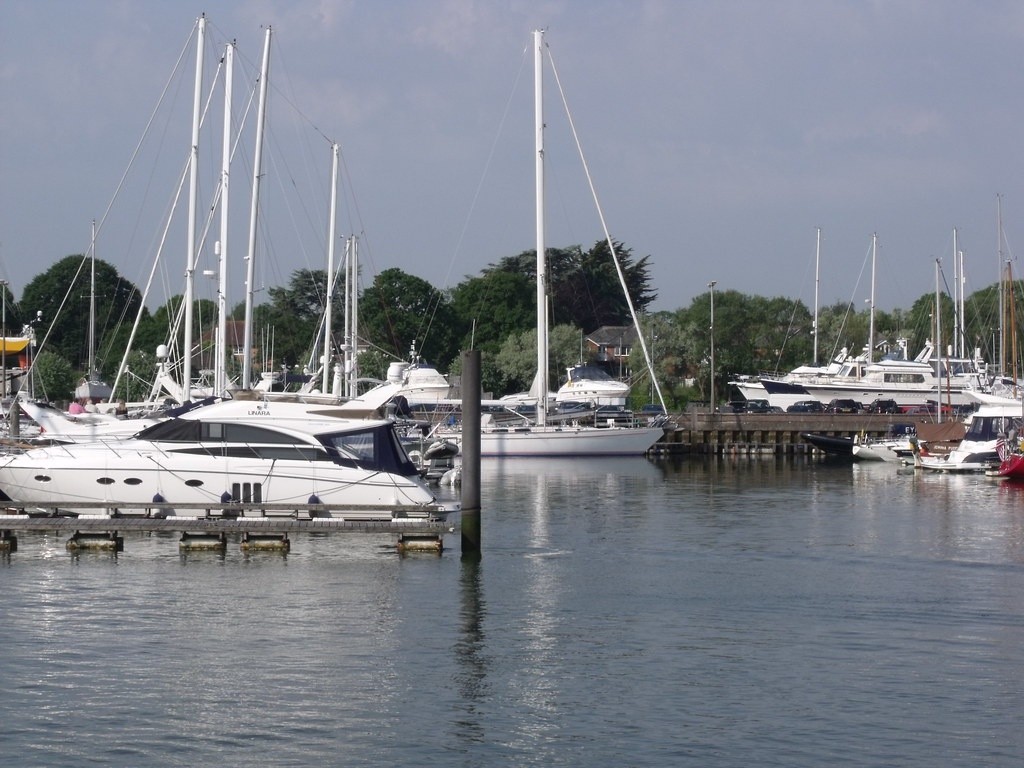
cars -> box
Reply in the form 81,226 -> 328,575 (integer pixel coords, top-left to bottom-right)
825,398 -> 859,414
866,398 -> 898,414
786,400 -> 822,412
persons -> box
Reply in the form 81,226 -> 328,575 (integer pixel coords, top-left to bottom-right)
112,399 -> 128,419
84,400 -> 99,413
69,399 -> 85,415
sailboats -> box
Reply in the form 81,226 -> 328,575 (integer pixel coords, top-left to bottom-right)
0,12 -> 1024,519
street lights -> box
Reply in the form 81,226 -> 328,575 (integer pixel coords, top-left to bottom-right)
707,279 -> 718,415
